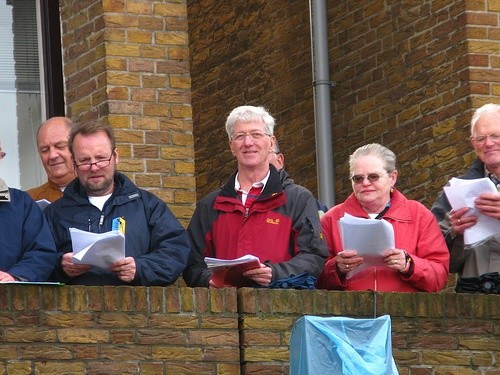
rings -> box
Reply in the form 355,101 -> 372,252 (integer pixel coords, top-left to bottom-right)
346,264 -> 348,268
395,260 -> 398,264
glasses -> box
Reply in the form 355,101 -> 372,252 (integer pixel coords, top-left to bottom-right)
349,169 -> 390,183
73,147 -> 116,171
474,131 -> 500,145
231,131 -> 271,142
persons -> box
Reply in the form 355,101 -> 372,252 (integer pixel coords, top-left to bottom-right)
431,103 -> 500,295
42,119 -> 192,287
0,179 -> 57,283
315,143 -> 450,292
182,106 -> 328,287
24,117 -> 78,211
